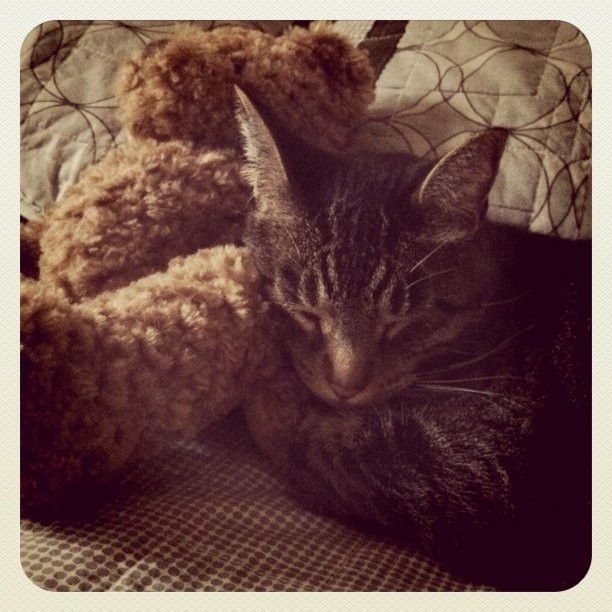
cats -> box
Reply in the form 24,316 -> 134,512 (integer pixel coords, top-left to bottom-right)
232,80 -> 591,581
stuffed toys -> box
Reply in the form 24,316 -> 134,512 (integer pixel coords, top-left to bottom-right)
20,23 -> 377,505
